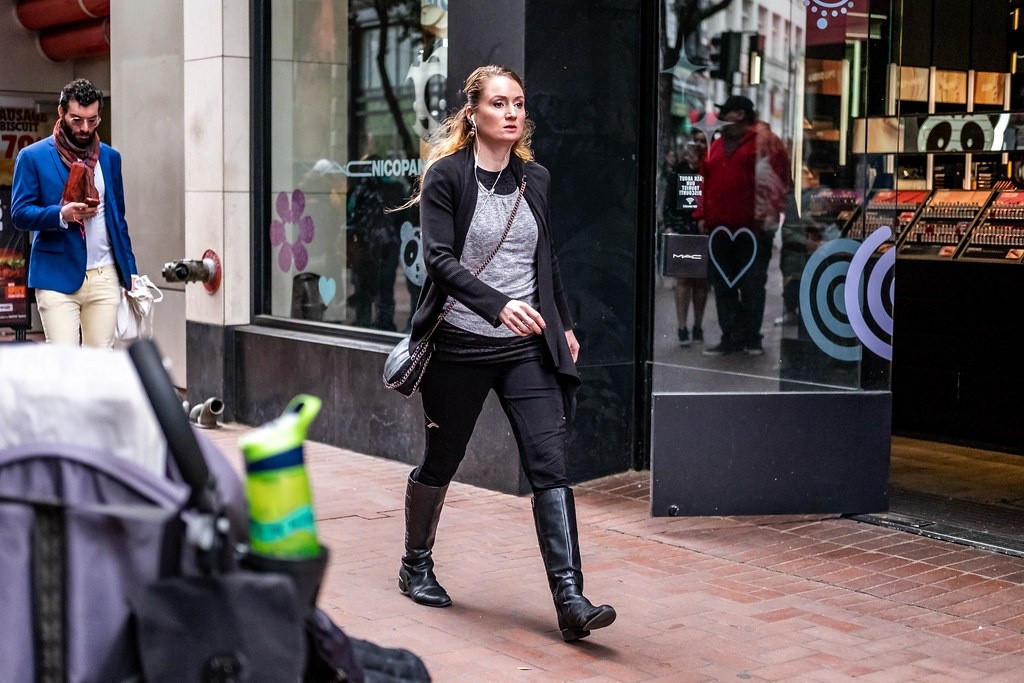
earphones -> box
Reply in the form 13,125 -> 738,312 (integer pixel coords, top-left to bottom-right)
471,113 -> 477,127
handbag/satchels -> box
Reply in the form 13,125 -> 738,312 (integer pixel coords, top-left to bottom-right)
117,275 -> 162,342
382,331 -> 436,400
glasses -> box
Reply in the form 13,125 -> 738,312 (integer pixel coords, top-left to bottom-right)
66,112 -> 101,128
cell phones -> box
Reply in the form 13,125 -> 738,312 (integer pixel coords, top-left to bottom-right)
84,197 -> 100,207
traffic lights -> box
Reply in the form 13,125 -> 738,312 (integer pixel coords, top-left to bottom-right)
681,116 -> 693,135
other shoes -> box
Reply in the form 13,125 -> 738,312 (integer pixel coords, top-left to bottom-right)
678,327 -> 690,344
703,343 -> 737,356
374,316 -> 397,330
744,342 -> 764,355
693,327 -> 702,343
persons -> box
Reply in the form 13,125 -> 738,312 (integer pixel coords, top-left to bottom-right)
664,96 -> 795,356
10,76 -> 142,349
399,67 -> 616,644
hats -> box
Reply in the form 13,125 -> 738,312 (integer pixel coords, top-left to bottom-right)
714,95 -> 753,112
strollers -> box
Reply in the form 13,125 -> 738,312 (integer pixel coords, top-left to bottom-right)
1,338 -> 433,683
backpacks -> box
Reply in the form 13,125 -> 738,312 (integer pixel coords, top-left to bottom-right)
107,490 -> 358,683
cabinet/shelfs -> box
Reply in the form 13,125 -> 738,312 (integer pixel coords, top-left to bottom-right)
801,188 -> 1024,456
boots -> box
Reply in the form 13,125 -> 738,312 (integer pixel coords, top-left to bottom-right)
530,488 -> 616,641
398,467 -> 452,607
